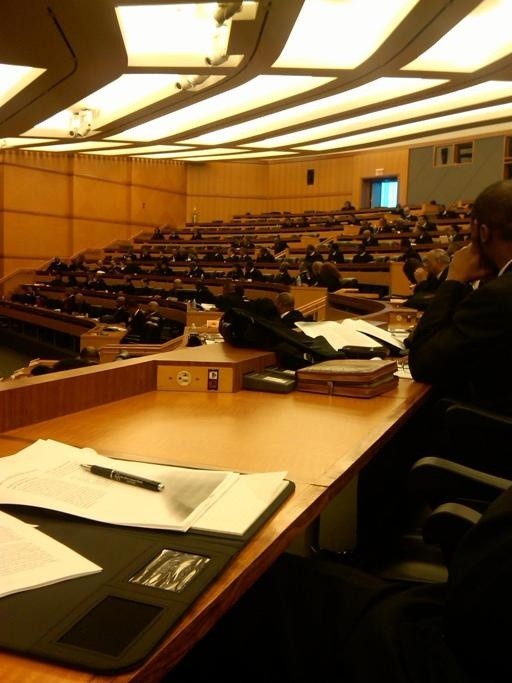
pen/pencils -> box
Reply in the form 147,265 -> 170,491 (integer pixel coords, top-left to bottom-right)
78,463 -> 165,492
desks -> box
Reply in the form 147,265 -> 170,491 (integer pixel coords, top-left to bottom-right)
0,345 -> 431,683
0,203 -> 478,364
0,356 -> 57,383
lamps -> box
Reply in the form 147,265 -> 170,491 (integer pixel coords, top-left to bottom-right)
175,0 -> 244,90
68,109 -> 93,138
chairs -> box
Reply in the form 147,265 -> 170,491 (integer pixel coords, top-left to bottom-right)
163,396 -> 512,683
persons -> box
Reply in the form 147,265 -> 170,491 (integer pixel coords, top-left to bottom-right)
307,179 -> 512,570
7,197 -> 478,380
